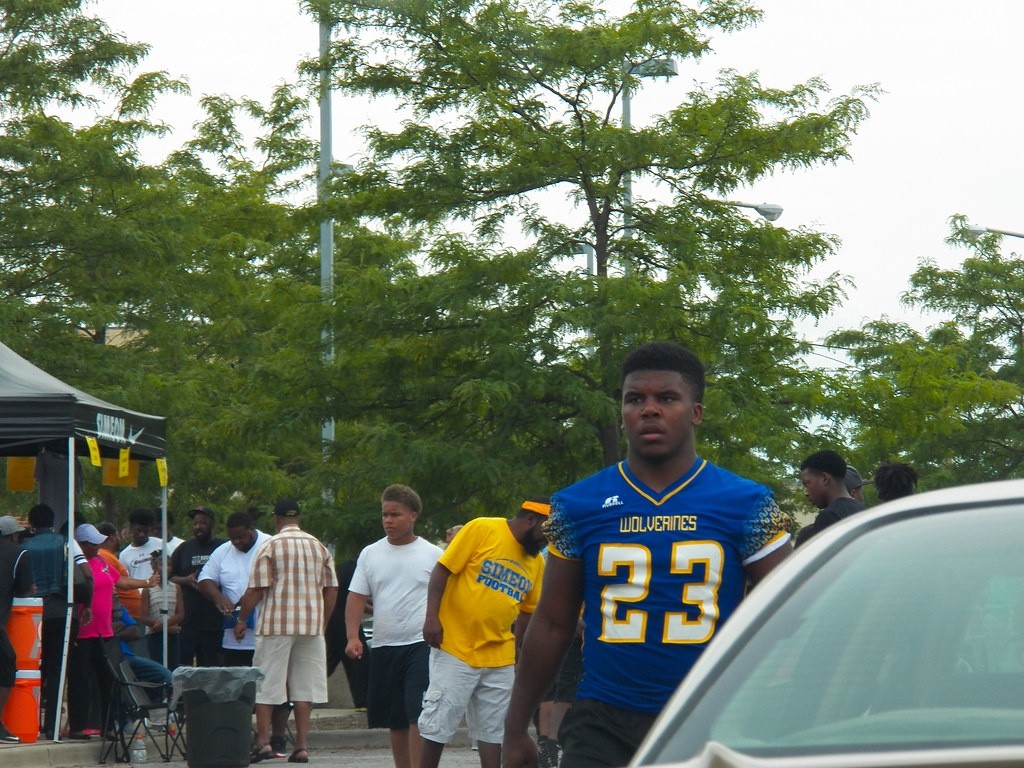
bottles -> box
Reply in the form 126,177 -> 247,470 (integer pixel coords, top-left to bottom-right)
169,720 -> 175,736
129,732 -> 148,768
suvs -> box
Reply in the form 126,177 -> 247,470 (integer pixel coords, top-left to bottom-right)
624,479 -> 1024,768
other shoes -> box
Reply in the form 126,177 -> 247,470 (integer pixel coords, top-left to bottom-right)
101,733 -> 118,742
70,733 -> 90,739
0,724 -> 20,744
269,736 -> 287,757
46,730 -> 62,740
123,721 -> 139,735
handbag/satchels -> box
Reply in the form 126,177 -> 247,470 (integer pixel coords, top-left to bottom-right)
223,602 -> 256,630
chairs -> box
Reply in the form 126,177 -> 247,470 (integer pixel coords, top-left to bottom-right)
251,677 -> 298,753
98,629 -> 187,763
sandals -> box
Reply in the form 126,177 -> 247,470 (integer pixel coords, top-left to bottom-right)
288,748 -> 308,763
250,743 -> 274,763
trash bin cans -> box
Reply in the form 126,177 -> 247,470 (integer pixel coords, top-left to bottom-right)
173,665 -> 265,768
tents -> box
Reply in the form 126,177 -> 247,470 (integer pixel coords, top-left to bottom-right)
0,342 -> 174,742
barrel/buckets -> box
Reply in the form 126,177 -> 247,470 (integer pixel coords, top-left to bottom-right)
0,596 -> 43,743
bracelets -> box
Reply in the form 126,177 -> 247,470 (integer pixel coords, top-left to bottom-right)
146,580 -> 150,588
236,617 -> 247,624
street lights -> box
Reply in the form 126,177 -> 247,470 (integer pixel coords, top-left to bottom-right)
621,56 -> 680,277
667,199 -> 785,276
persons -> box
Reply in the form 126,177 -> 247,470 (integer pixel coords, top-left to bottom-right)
875,462 -> 920,502
1,483 -> 588,768
502,342 -> 792,768
793,450 -> 871,548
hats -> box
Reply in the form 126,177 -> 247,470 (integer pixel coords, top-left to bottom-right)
75,524 -> 108,544
0,516 -> 26,536
844,465 -> 875,493
272,499 -> 300,516
187,507 -> 215,520
